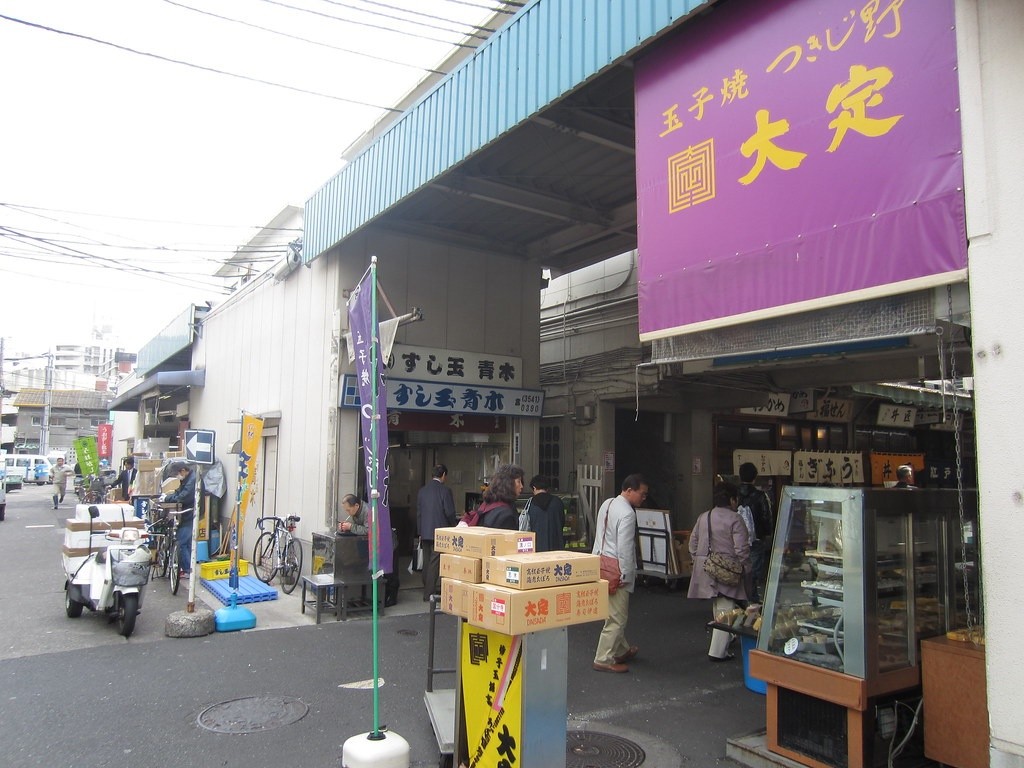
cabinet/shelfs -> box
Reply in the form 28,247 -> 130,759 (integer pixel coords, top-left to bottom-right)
749,485 -> 982,768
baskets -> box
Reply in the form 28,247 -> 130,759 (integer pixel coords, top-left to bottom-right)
110,545 -> 152,586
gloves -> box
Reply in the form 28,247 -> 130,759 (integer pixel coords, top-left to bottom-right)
159,492 -> 167,503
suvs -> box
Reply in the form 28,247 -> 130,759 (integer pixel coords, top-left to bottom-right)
0,449 -> 8,520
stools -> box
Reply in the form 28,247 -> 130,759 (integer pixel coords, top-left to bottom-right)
301,574 -> 343,624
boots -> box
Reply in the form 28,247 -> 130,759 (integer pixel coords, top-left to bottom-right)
59,493 -> 65,503
53,495 -> 58,509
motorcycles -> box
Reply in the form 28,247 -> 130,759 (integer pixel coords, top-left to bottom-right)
62,504 -> 167,636
73,474 -> 84,496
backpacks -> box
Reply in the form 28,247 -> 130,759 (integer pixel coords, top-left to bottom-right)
455,500 -> 512,527
736,492 -> 756,545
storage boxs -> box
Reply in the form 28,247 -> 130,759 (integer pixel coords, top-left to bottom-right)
137,459 -> 163,472
62,503 -> 146,575
199,559 -> 249,581
434,526 -> 610,636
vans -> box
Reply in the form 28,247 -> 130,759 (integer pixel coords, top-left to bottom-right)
6,454 -> 54,486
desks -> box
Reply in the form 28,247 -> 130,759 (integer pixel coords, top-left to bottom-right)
921,634 -> 991,768
325,578 -> 389,616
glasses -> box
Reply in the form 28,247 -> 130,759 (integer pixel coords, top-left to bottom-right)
631,489 -> 648,497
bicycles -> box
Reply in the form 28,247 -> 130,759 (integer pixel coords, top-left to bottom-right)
252,514 -> 303,595
80,473 -> 111,504
146,498 -> 193,595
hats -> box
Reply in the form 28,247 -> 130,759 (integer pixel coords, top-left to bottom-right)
169,461 -> 186,477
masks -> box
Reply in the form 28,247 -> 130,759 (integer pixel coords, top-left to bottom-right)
176,472 -> 186,481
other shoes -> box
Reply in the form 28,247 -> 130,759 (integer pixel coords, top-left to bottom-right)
729,632 -> 737,645
179,570 -> 189,579
709,652 -> 733,662
385,591 -> 396,607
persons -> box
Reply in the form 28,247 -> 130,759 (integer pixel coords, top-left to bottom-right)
108,457 -> 138,502
475,464 -> 524,531
417,463 -> 458,601
591,474 -> 649,674
522,474 -> 566,552
891,464 -> 918,489
50,458 -> 75,509
686,481 -> 754,662
734,463 -> 775,602
159,461 -> 205,579
339,493 -> 400,608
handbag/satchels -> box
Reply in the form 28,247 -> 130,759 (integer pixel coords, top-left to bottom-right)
518,497 -> 534,532
703,553 -> 743,587
407,541 -> 423,575
599,554 -> 621,595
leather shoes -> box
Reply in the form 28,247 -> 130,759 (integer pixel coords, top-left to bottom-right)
592,661 -> 630,673
613,644 -> 638,662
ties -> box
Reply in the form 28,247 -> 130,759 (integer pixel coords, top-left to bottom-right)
128,470 -> 130,486
